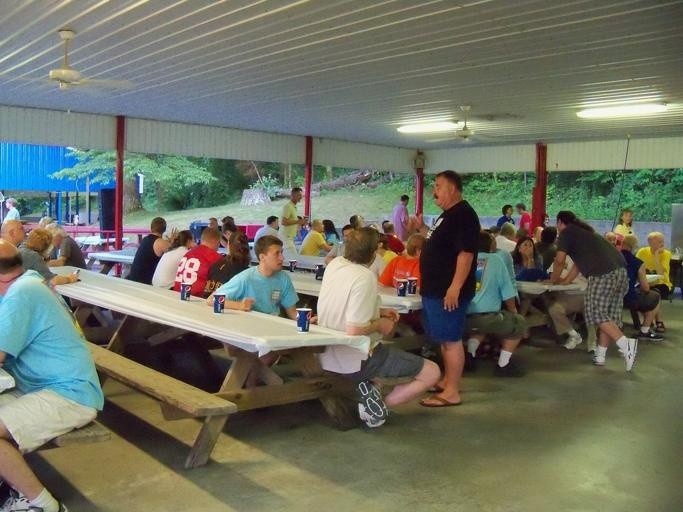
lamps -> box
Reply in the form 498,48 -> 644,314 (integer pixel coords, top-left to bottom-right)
571,99 -> 670,121
393,116 -> 460,136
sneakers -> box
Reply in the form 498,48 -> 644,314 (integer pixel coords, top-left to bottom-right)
464,351 -> 483,369
0,488 -> 68,512
496,362 -> 525,377
617,337 -> 639,372
636,328 -> 663,341
592,355 -> 606,366
563,332 -> 582,350
656,322 -> 665,333
358,402 -> 386,429
352,373 -> 388,420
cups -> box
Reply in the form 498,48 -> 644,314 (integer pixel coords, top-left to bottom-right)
407,278 -> 417,296
314,264 -> 323,280
295,307 -> 312,334
180,282 -> 191,301
396,279 -> 407,296
288,259 -> 296,272
212,293 -> 226,314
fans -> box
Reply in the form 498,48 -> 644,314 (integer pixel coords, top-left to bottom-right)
1,29 -> 136,92
423,103 -> 501,151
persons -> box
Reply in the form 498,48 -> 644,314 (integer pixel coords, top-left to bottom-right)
1,240 -> 104,512
542,209 -> 639,370
316,228 -> 442,429
1,198 -> 84,308
421,167 -> 480,408
494,205 -> 515,231
612,210 -> 636,238
391,195 -> 412,243
514,202 -> 534,232
464,222 -> 671,378
295,216 -> 422,290
280,187 -> 305,246
130,215 -> 301,390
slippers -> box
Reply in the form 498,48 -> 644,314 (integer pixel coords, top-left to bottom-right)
418,394 -> 462,407
426,383 -> 461,393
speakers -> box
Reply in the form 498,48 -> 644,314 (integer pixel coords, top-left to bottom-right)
98,188 -> 116,239
